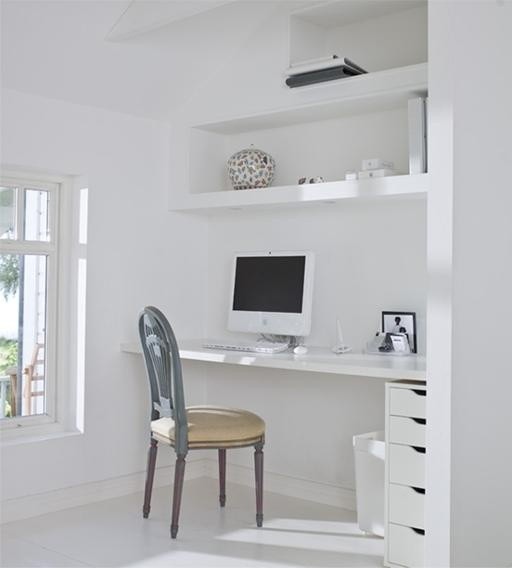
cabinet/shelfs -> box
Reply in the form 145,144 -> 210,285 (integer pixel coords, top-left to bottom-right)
167,0 -> 433,211
382,379 -> 427,568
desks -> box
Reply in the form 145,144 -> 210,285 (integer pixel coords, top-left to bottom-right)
121,333 -> 427,383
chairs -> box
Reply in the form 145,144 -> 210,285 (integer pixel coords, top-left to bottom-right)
138,304 -> 268,540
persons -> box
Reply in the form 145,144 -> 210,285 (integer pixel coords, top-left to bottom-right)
392,316 -> 402,332
398,327 -> 410,342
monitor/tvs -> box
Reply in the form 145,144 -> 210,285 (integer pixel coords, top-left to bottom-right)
227,250 -> 314,348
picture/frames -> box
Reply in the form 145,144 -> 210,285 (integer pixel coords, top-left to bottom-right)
381,309 -> 417,353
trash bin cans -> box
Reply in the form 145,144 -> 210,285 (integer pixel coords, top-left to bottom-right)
353,431 -> 384,539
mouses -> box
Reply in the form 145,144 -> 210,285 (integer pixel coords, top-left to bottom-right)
294,345 -> 308,355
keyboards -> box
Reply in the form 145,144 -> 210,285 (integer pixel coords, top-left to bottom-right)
202,341 -> 288,355
